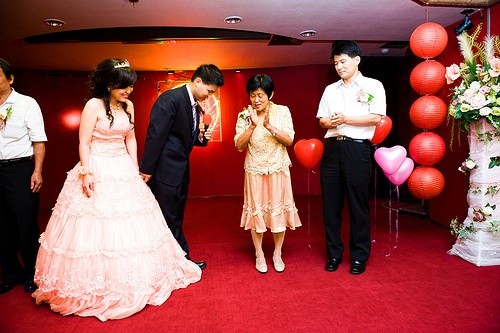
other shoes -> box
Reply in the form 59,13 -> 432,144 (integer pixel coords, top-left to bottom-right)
256,256 -> 267,272
273,254 -> 286,271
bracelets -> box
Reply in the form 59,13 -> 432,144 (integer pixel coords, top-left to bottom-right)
273,128 -> 279,136
77,166 -> 93,175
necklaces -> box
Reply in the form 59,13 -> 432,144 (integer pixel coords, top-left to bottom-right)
110,102 -> 121,111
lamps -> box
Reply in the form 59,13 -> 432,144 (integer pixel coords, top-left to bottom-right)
454,9 -> 476,35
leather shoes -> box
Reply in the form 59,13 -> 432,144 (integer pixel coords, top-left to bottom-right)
351,260 -> 364,274
195,261 -> 206,269
0,281 -> 16,293
325,257 -> 341,270
25,278 -> 36,291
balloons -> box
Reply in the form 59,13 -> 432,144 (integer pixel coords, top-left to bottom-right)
374,146 -> 407,174
383,156 -> 415,185
293,138 -> 323,172
369,116 -> 393,145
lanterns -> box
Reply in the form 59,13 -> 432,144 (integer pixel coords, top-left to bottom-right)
407,6 -> 448,199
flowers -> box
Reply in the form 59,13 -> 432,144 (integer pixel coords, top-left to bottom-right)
237,106 -> 251,125
356,90 -> 374,105
0,105 -> 13,126
444,23 -> 500,240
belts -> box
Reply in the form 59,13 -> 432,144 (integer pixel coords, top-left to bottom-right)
0,157 -> 29,164
329,136 -> 368,144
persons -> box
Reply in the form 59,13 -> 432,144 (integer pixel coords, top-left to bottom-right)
234,74 -> 302,274
0,59 -> 48,294
31,58 -> 202,320
316,40 -> 387,273
140,64 -> 224,269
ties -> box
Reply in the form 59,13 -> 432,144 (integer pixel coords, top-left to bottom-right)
192,102 -> 197,132
